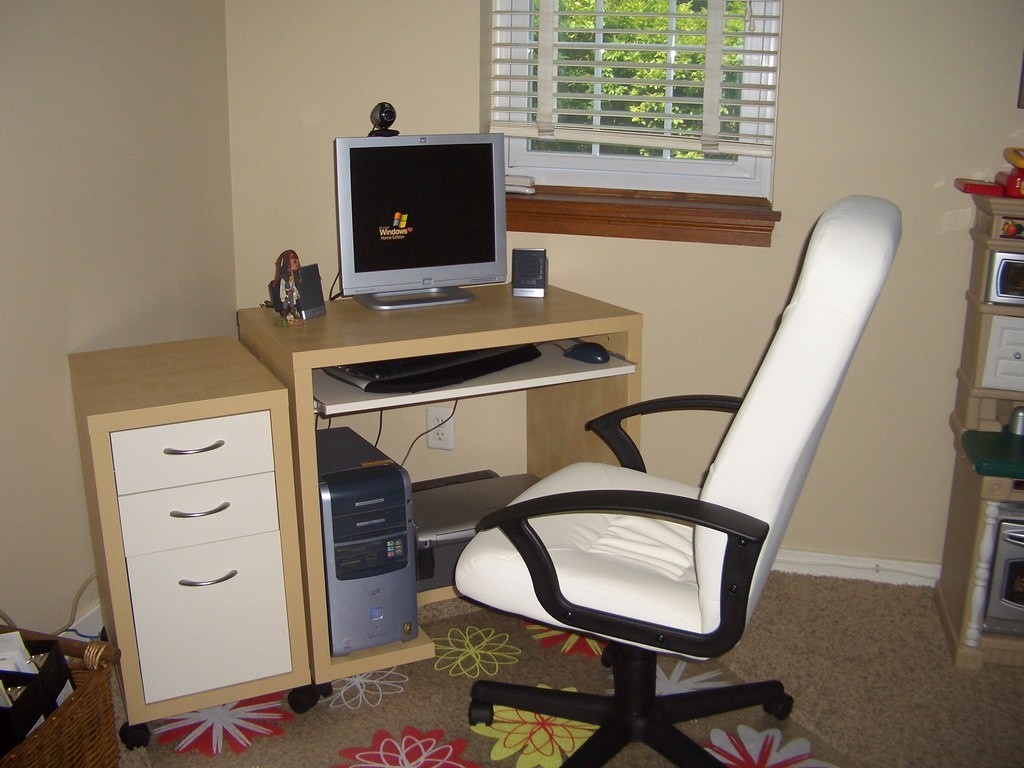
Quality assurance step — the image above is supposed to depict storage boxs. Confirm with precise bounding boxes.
[0,639,80,761]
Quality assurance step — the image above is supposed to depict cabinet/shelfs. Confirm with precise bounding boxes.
[68,334,311,750]
[933,172,1024,671]
[239,283,643,697]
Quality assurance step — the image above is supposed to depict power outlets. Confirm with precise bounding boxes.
[426,407,453,450]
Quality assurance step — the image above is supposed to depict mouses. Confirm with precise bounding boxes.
[563,342,611,364]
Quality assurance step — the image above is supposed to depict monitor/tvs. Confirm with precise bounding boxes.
[336,133,507,310]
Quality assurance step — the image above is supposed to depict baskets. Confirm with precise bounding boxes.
[0,662,120,768]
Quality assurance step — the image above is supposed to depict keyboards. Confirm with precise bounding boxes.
[323,343,542,394]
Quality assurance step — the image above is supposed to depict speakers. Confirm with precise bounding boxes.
[512,248,548,297]
[295,263,326,320]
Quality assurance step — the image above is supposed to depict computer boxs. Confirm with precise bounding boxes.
[316,426,418,657]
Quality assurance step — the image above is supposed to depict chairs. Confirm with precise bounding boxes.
[456,195,903,768]
